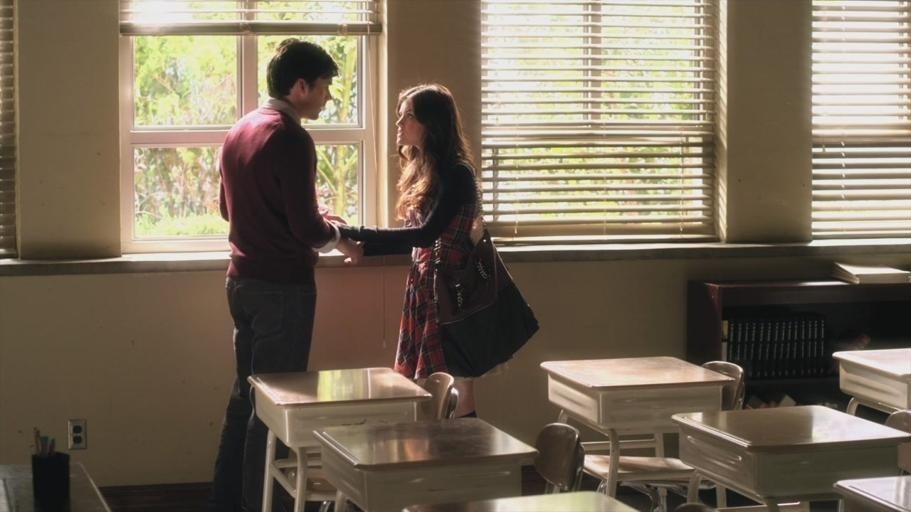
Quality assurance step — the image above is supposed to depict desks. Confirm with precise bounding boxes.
[670,405,911,511]
[832,348,910,416]
[0,460,110,511]
[832,475,911,511]
[248,367,433,512]
[400,490,641,512]
[314,418,539,512]
[539,356,736,508]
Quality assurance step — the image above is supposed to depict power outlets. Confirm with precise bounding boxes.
[68,418,87,450]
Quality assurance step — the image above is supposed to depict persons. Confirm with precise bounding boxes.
[210,38,361,509]
[332,85,539,423]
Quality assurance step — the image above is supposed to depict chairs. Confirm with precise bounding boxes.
[535,423,584,494]
[584,360,746,512]
[291,372,461,511]
[883,410,911,475]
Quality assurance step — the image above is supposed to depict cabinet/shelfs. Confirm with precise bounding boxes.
[686,277,911,431]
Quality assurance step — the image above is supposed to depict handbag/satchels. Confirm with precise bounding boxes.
[432,158,499,326]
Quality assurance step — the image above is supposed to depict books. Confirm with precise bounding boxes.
[825,262,909,283]
